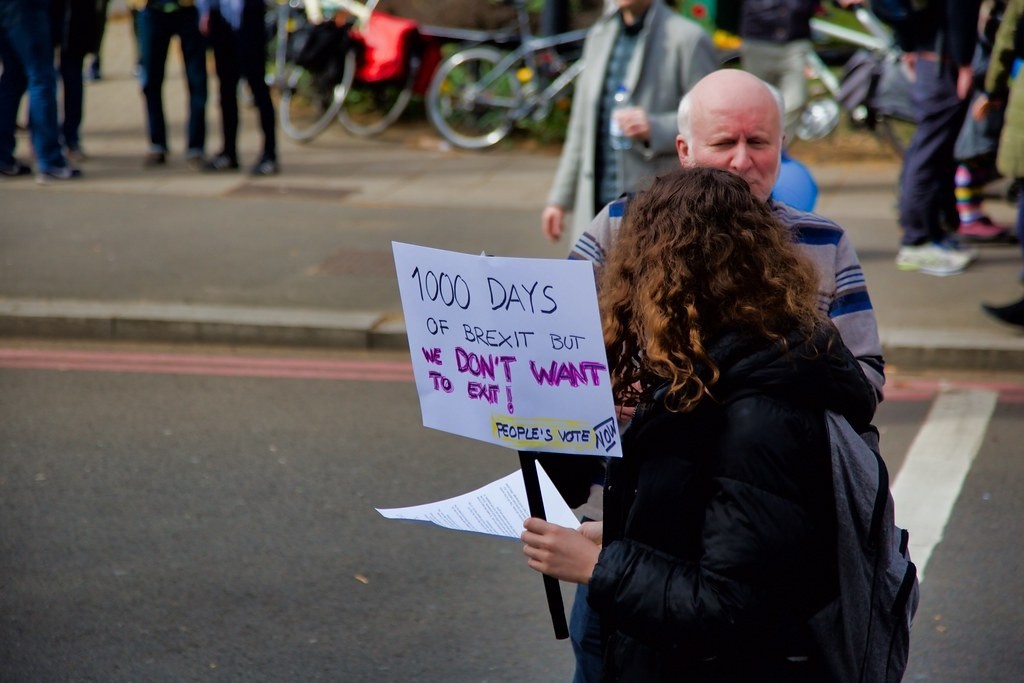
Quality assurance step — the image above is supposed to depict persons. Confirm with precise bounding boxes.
[0,0,281,184]
[490,0,1024,683]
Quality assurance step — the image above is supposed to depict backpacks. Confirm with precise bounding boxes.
[814,410,919,683]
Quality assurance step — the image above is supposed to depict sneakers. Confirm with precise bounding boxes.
[895,241,970,273]
[0,160,32,178]
[37,166,80,183]
[941,236,978,259]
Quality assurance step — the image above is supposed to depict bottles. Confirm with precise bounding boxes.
[609,86,634,152]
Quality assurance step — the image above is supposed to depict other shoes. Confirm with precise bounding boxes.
[186,153,206,170]
[87,63,98,80]
[65,147,91,164]
[981,298,1024,329]
[145,152,164,166]
[252,159,277,175]
[207,153,238,170]
[957,217,1008,238]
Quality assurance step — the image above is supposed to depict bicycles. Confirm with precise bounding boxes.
[266,1,594,151]
[800,7,924,160]
